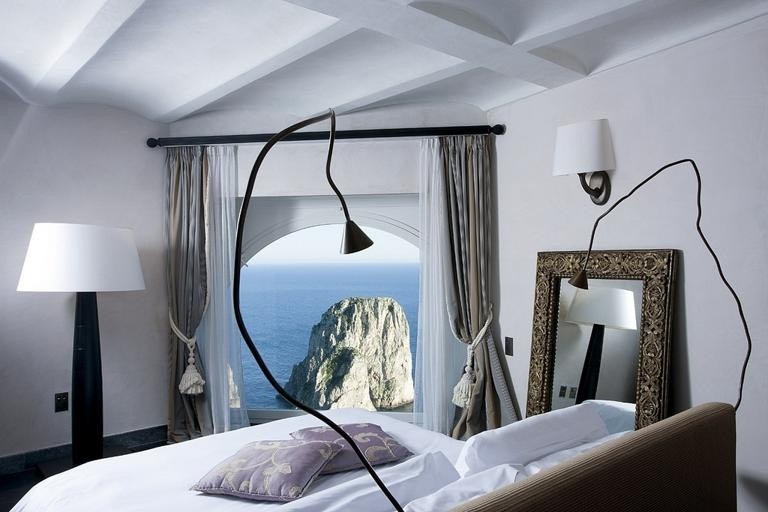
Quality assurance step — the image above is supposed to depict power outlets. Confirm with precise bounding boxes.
[54,392,69,413]
[559,385,567,397]
[569,387,577,398]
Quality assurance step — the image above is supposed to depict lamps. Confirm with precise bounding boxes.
[233,107,403,512]
[565,286,638,405]
[16,223,146,466]
[570,157,751,411]
[550,117,616,206]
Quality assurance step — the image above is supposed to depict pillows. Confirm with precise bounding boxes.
[272,451,460,512]
[291,423,413,476]
[454,401,608,477]
[522,429,634,476]
[404,463,532,512]
[187,441,343,503]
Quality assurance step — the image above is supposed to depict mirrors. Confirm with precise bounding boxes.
[526,249,679,431]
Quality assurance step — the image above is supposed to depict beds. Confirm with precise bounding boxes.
[12,401,738,511]
[583,398,636,432]
